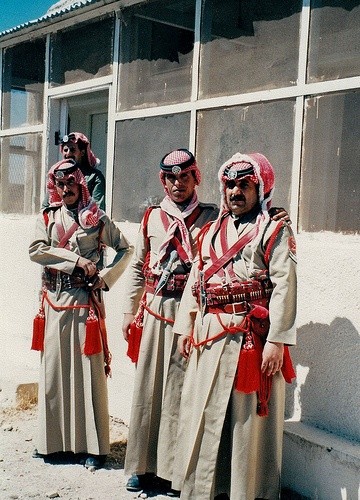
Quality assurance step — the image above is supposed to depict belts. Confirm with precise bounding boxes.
[205,298,269,315]
[146,284,183,298]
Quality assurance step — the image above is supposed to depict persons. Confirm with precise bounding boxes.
[121,147,222,492]
[41,133,105,214]
[172,152,297,500]
[28,160,134,467]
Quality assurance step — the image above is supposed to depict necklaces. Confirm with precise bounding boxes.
[69,208,78,211]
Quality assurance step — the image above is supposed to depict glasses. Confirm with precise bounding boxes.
[56,178,75,187]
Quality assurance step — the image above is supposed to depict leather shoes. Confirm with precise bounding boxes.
[31,452,41,458]
[85,456,99,468]
[125,474,141,490]
